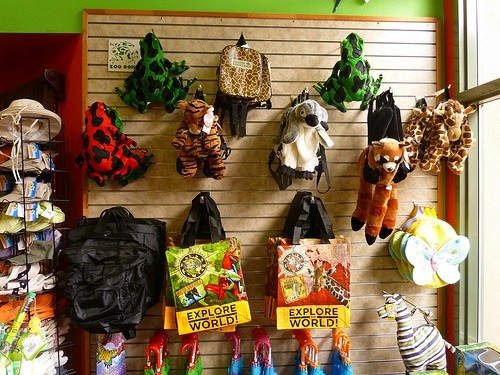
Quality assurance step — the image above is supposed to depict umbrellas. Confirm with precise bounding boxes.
[143,328,353,375]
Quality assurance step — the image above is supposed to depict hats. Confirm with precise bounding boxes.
[0,100,64,264]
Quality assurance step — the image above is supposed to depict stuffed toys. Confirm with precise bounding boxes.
[171,100,226,182]
[116,33,191,113]
[278,100,328,180]
[352,138,406,247]
[313,32,383,112]
[378,293,446,375]
[405,100,476,176]
[77,102,139,188]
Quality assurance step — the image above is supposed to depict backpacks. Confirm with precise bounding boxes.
[215,36,272,136]
[267,92,334,193]
[171,88,231,180]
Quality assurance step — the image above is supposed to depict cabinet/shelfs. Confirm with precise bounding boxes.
[0,68,76,375]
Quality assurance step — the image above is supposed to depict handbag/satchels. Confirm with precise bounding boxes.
[165,192,251,336]
[454,341,500,375]
[60,207,166,334]
[264,190,350,331]
